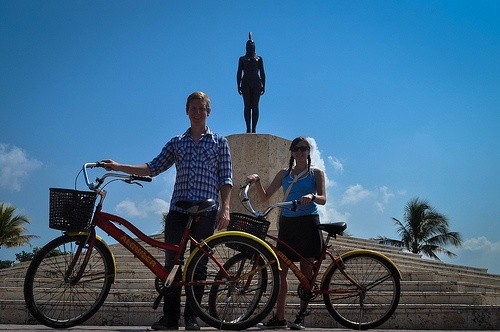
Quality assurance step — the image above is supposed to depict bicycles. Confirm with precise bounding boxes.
[23,161,283,330]
[207,177,403,330]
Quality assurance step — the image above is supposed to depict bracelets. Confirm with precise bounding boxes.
[311,193,316,201]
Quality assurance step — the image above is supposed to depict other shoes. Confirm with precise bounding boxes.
[263,317,287,328]
[291,312,305,329]
[151,315,179,329]
[185,317,200,330]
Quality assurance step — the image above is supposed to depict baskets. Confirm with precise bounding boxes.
[224,212,271,257]
[49,187,97,232]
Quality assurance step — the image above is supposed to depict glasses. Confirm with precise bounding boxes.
[289,146,308,151]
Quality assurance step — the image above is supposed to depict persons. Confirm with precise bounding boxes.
[101,92,233,330]
[247,136,326,330]
[236,32,265,133]
